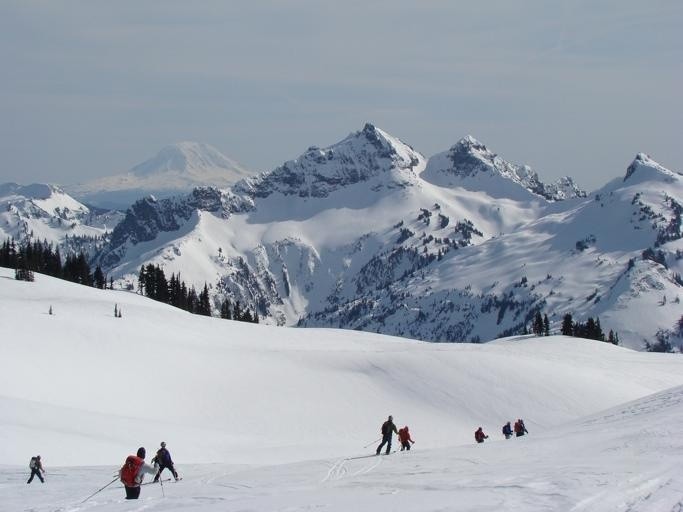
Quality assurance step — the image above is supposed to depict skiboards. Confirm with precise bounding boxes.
[145,478,187,485]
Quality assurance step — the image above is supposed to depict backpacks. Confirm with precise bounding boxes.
[120,455,144,488]
[29,457,38,469]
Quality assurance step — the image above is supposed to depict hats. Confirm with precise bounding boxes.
[137,447,145,458]
[387,415,526,442]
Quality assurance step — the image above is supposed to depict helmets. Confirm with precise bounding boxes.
[161,442,166,447]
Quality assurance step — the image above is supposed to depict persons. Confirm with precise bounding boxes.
[27,455,45,486]
[503,420,513,439]
[397,426,416,452]
[152,442,181,484]
[375,416,398,455]
[118,446,161,500]
[474,425,489,445]
[514,419,529,438]
